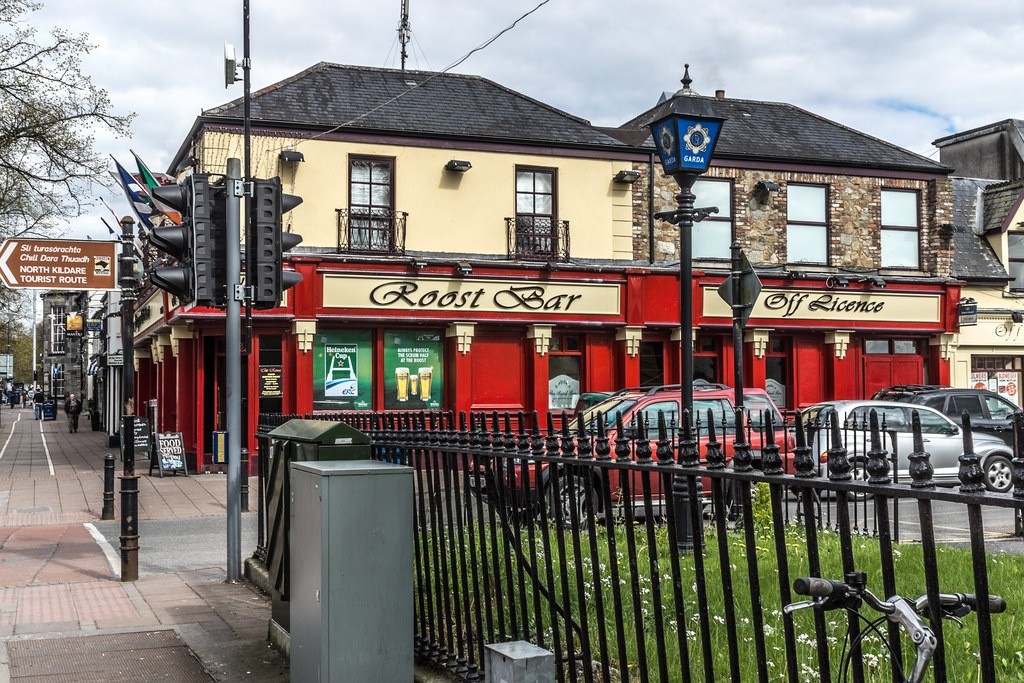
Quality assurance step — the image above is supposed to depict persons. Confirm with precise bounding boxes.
[64,394,82,434]
[4,386,44,420]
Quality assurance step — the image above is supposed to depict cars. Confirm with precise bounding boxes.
[872,384,1022,482]
[780,400,1012,503]
[467,383,799,533]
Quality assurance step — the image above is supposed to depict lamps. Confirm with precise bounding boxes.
[873,277,888,286]
[410,257,429,269]
[791,269,809,278]
[455,261,473,273]
[1011,311,1024,323]
[939,222,957,232]
[446,159,472,171]
[278,151,306,162]
[544,262,561,272]
[832,275,849,286]
[611,170,640,183]
[752,180,783,193]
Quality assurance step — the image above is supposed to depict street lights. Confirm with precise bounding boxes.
[647,59,728,555]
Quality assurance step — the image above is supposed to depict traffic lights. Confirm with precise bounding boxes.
[250,173,304,311]
[148,178,220,312]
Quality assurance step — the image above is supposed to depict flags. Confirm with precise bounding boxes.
[110,156,183,260]
